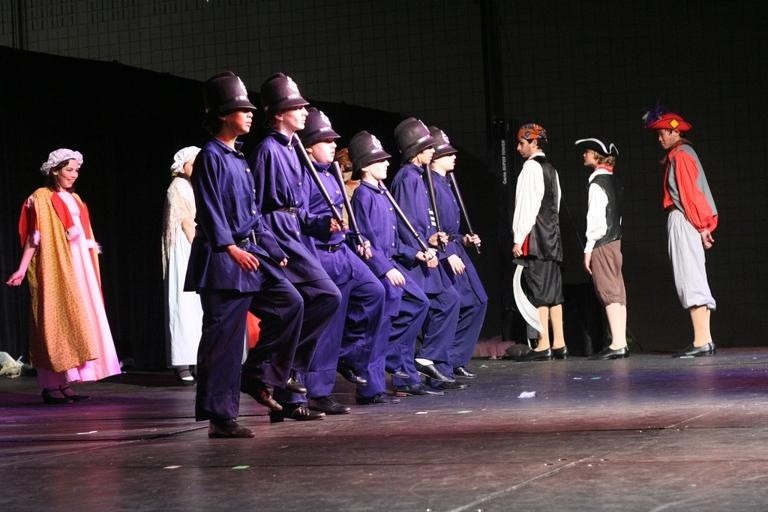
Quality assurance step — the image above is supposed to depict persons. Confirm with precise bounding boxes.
[512,123,568,362]
[5,148,122,406]
[574,131,630,360]
[642,112,720,359]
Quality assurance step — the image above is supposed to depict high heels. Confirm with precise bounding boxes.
[174,367,195,387]
[61,385,92,402]
[41,388,74,404]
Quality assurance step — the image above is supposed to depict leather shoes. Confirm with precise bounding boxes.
[239,367,285,412]
[267,404,327,420]
[286,377,307,393]
[308,394,352,414]
[334,353,478,405]
[518,346,719,360]
[208,419,255,439]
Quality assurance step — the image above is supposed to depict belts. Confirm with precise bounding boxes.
[232,239,250,248]
[447,235,456,241]
[276,206,299,216]
[316,241,343,252]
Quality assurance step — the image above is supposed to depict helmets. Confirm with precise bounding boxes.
[348,128,390,181]
[394,116,438,161]
[260,72,310,120]
[426,126,456,157]
[298,106,340,147]
[203,71,258,130]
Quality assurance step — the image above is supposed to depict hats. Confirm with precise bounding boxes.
[575,123,620,157]
[643,112,692,132]
[40,147,84,176]
[172,146,200,173]
